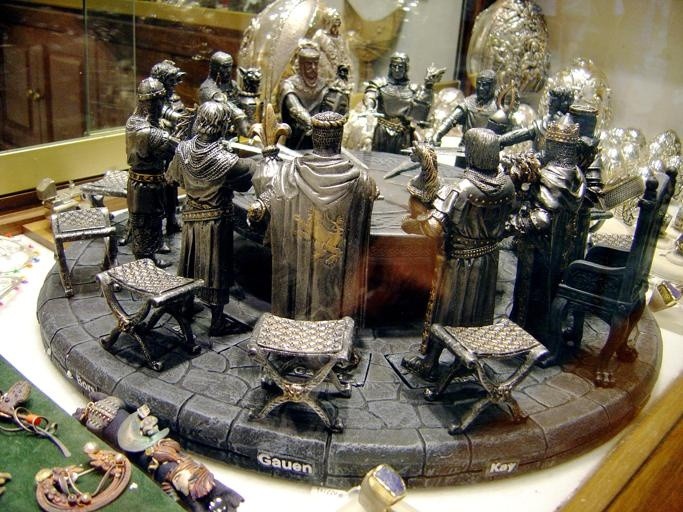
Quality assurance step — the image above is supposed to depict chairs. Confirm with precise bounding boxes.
[538,167,678,389]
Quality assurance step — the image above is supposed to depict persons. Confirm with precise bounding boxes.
[126,8,606,382]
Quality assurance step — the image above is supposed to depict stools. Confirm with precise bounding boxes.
[79,170,132,246]
[247,312,355,434]
[51,207,122,298]
[422,315,551,435]
[95,257,205,372]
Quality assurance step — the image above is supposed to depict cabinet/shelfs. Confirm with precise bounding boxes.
[0,0,259,153]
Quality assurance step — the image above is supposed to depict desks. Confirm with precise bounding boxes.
[231,149,466,325]
